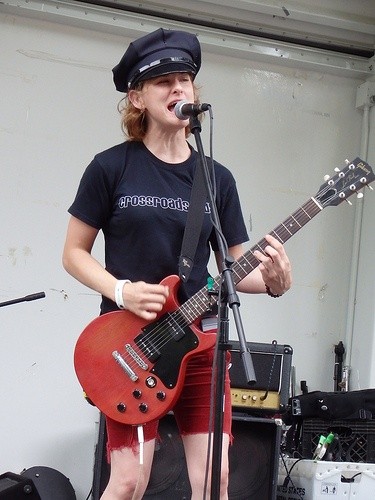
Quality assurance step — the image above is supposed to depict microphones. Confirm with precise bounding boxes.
[173,101,211,120]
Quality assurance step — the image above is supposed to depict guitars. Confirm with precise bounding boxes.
[74,156,375,428]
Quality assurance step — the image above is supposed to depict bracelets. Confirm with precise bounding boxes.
[264,284,283,297]
[115,279,130,309]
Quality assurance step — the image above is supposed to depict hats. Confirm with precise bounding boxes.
[112,27,201,93]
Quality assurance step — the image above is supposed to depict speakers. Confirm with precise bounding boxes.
[227,340,294,413]
[91,410,283,500]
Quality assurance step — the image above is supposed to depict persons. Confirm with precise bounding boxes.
[63,28,292,500]
